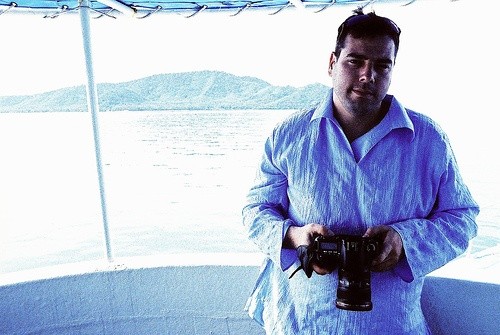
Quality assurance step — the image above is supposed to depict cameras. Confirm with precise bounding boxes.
[313,235,382,311]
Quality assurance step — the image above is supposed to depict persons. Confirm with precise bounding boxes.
[233,6,481,335]
